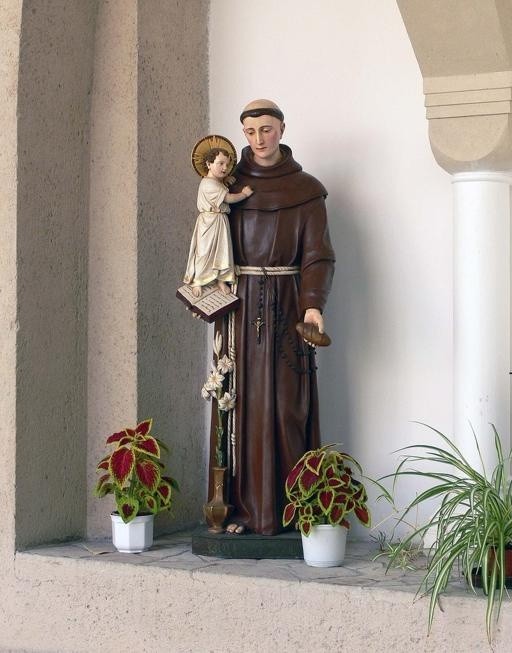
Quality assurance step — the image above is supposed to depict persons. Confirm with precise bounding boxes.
[183,148,254,300]
[185,97,337,534]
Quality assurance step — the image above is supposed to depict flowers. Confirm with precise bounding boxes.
[196,331,251,468]
[280,438,374,537]
[90,419,182,525]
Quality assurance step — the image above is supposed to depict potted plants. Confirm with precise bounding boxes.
[371,423,512,651]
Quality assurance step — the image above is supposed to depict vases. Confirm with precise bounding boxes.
[298,522,349,568]
[111,511,154,548]
[202,468,235,533]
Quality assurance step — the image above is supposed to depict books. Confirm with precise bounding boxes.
[176,280,240,322]
